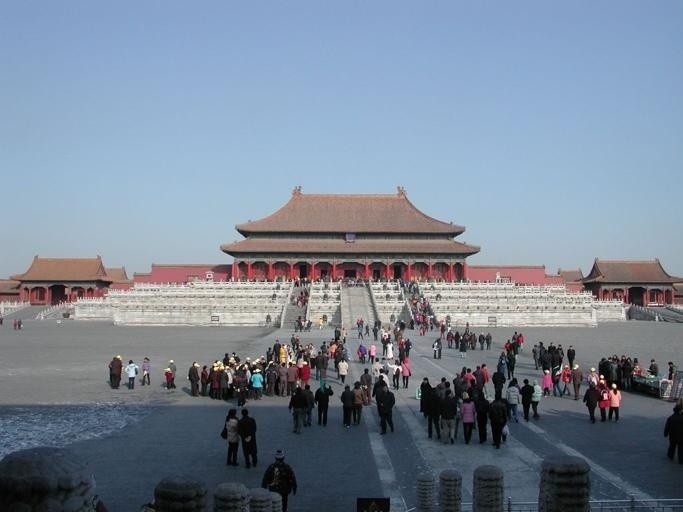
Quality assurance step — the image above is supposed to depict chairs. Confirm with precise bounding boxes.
[343,423,350,429]
[227,462,252,468]
[450,438,455,444]
[379,428,395,435]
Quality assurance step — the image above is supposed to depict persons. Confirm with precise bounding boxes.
[14,319,18,330]
[107,272,683,468]
[262,449,297,512]
[18,319,21,329]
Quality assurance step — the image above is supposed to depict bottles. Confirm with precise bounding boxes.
[163,368,170,371]
[532,379,538,384]
[611,383,617,389]
[273,449,284,460]
[192,352,310,374]
[543,369,549,373]
[590,367,596,371]
[167,359,174,363]
[599,375,605,385]
[573,364,579,369]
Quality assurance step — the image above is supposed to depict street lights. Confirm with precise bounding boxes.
[500,424,509,442]
[408,369,411,376]
[220,428,228,440]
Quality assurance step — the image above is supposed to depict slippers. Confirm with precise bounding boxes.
[599,389,608,400]
[394,367,400,377]
